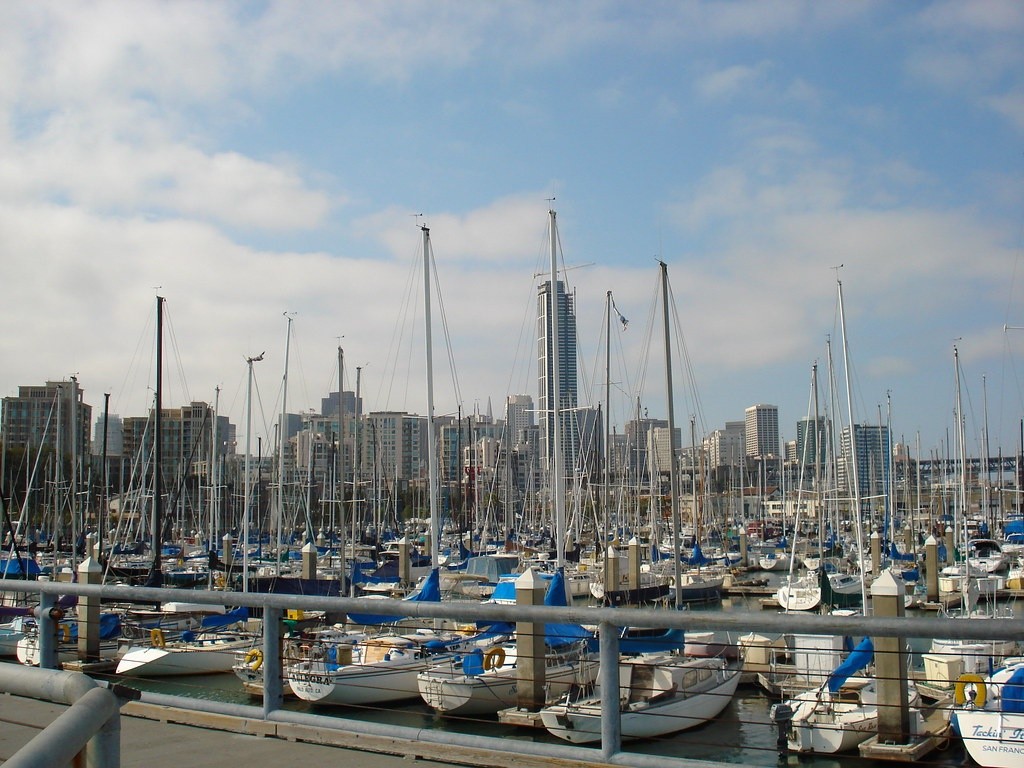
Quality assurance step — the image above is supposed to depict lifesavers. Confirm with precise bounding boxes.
[482,647,506,670]
[151,629,165,648]
[954,674,987,708]
[58,624,70,642]
[245,649,263,671]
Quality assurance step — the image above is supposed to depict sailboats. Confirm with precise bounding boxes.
[0,194,1024,767]
